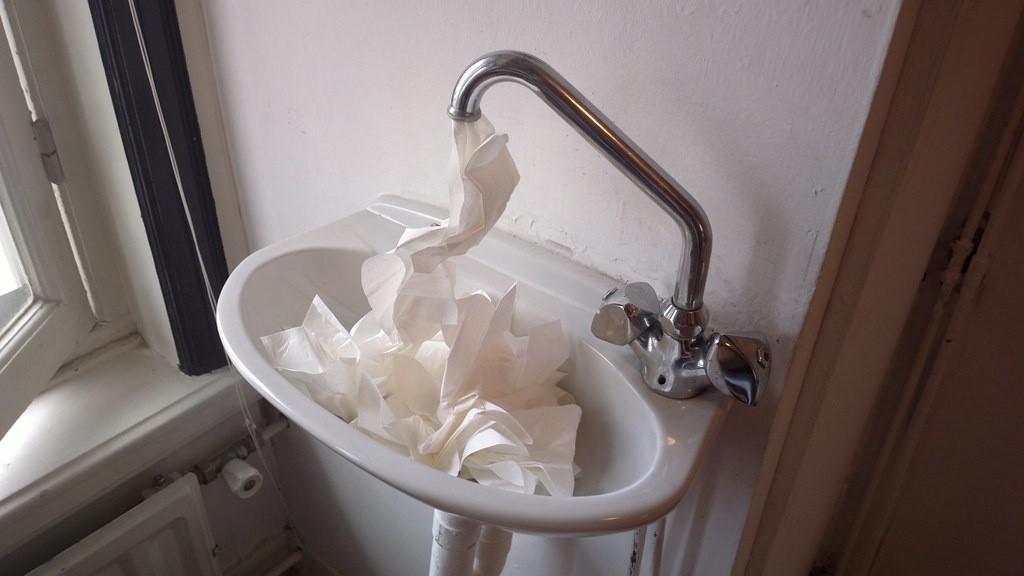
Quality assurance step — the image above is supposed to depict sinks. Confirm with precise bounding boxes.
[215,204,732,540]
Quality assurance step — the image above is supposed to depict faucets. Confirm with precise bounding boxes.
[443,50,711,329]
